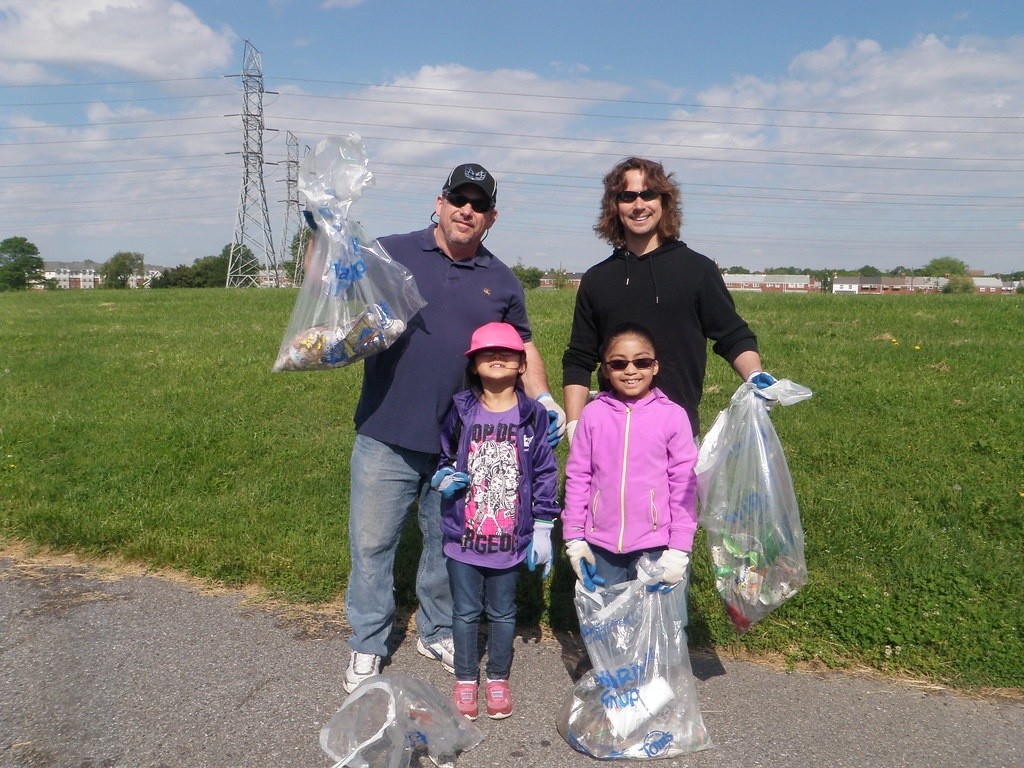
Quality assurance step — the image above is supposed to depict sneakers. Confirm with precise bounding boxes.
[417,637,456,675]
[453,679,478,721]
[342,648,381,693]
[484,677,513,719]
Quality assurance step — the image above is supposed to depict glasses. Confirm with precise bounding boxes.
[617,188,658,202]
[442,191,494,213]
[604,357,655,370]
[476,347,522,356]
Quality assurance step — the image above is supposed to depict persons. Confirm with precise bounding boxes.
[304,163,567,695]
[561,322,697,607]
[559,154,778,680]
[429,320,561,721]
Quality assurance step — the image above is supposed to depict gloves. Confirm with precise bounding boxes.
[303,188,335,231]
[536,393,566,450]
[746,371,778,414]
[646,548,689,593]
[431,467,470,500]
[523,518,554,578]
[565,539,605,592]
[567,420,578,447]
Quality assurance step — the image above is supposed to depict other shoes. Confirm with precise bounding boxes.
[573,655,593,680]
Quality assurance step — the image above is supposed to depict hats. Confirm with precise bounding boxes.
[442,163,498,208]
[465,322,526,357]
